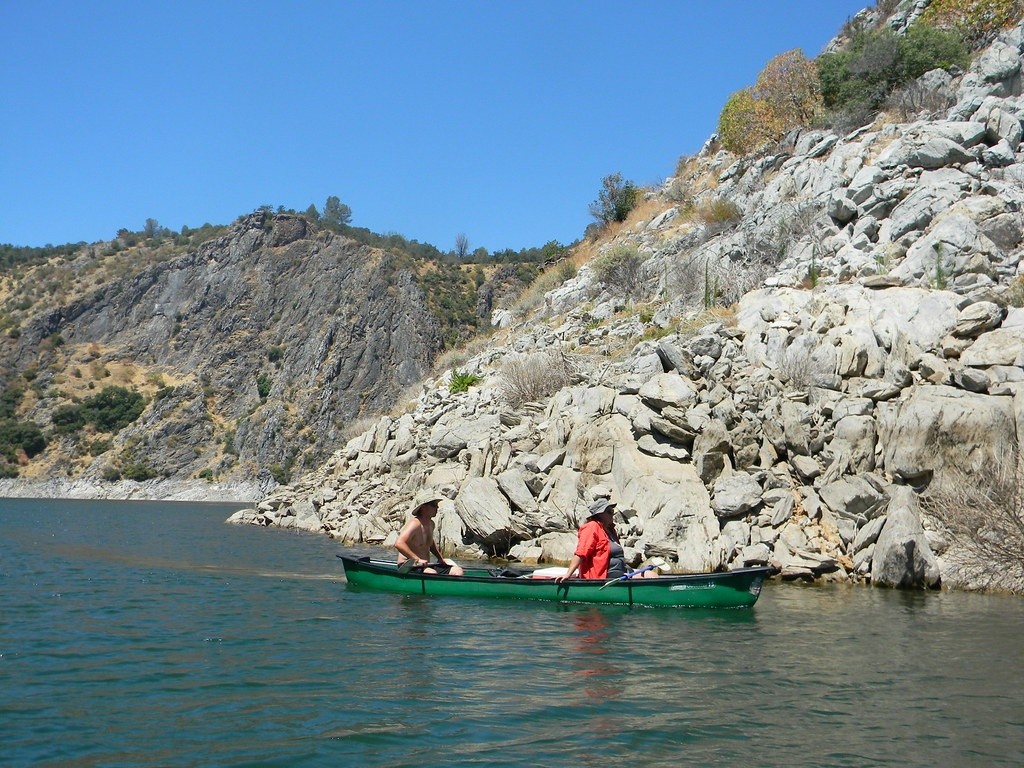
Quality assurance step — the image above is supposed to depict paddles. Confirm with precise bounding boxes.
[396,558,476,575]
[598,557,672,591]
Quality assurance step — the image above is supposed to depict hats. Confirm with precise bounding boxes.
[586,498,617,519]
[412,488,443,515]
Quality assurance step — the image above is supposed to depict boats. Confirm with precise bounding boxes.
[335,553,782,612]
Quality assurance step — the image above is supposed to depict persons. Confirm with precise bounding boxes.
[554,484,625,583]
[395,493,463,578]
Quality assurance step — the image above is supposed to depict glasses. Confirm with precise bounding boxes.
[427,502,439,508]
[605,509,614,515]
